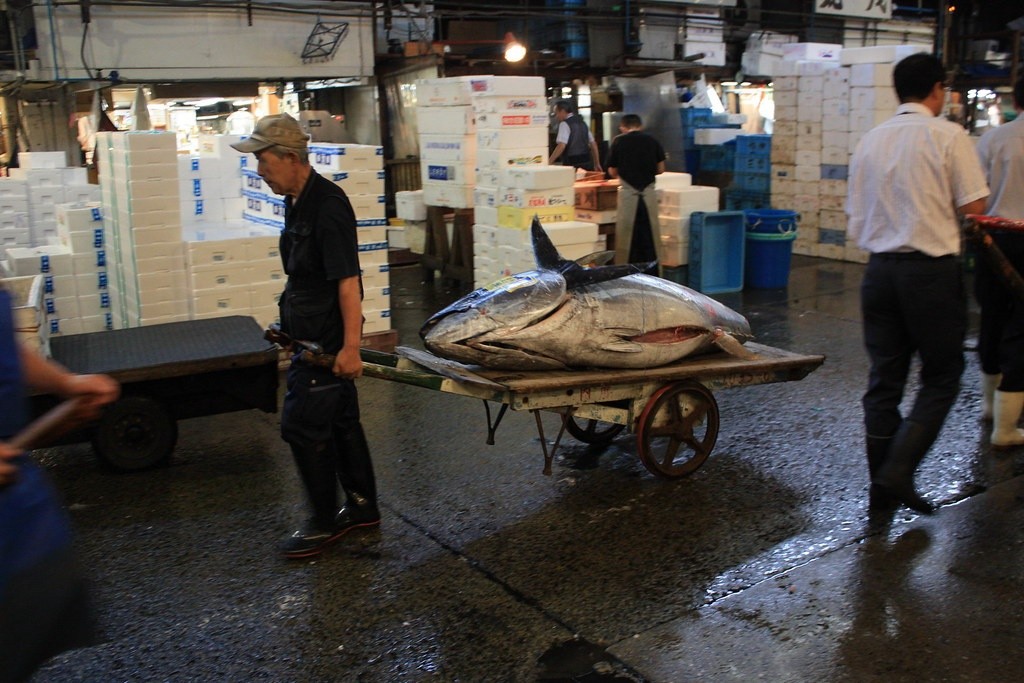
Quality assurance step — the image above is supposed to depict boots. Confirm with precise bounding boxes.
[875,420,935,516]
[990,387,1024,443]
[979,371,1002,419]
[865,432,896,525]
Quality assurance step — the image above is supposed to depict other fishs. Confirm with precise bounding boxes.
[418,212,754,371]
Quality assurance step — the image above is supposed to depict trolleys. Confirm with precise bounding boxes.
[263,320,826,487]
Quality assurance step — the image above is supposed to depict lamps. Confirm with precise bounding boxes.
[503,31,527,63]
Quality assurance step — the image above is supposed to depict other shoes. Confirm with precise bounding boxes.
[278,498,381,556]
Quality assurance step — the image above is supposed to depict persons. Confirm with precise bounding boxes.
[230,113,385,556]
[973,74,1024,446]
[604,114,666,277]
[846,52,990,530]
[548,101,603,171]
[0,288,118,683]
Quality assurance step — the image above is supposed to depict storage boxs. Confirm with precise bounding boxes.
[385,75,620,293]
[0,131,391,360]
[635,2,950,294]
[542,0,590,59]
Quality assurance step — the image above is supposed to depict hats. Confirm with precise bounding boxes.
[229,113,310,153]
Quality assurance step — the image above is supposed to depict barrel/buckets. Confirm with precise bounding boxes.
[742,206,800,289]
